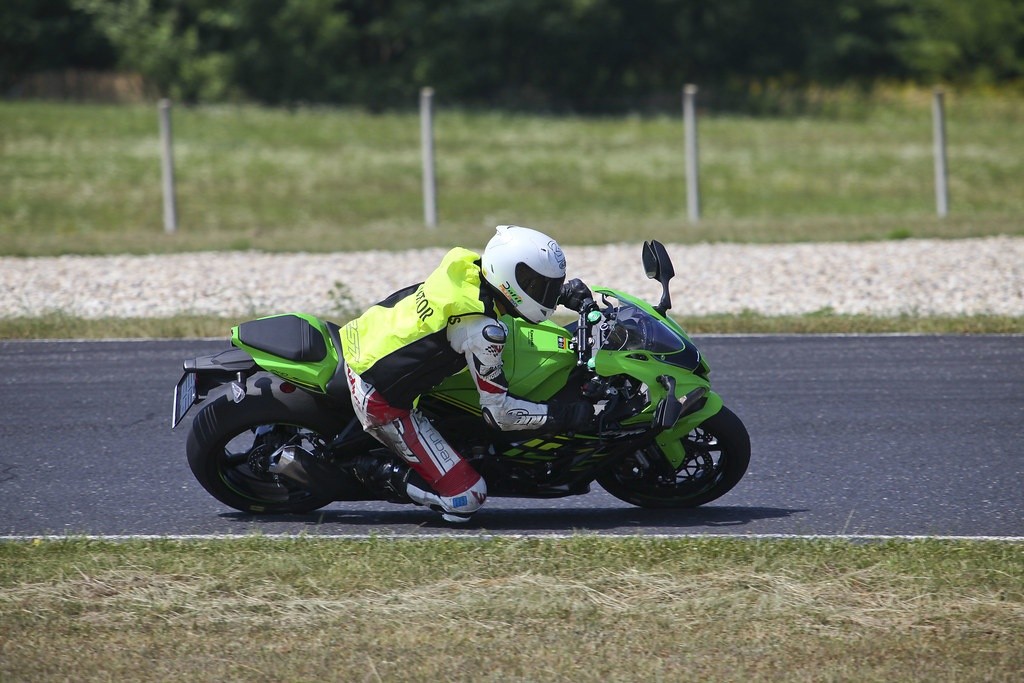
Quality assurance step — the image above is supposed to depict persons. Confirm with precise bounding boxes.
[339,225,593,523]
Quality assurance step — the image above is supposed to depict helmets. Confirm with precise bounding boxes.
[482,224,567,325]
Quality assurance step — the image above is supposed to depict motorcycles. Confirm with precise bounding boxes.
[173,239,752,518]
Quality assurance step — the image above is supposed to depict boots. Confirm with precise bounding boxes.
[347,442,424,506]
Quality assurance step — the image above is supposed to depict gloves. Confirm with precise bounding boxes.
[556,277,594,312]
[535,398,595,433]
